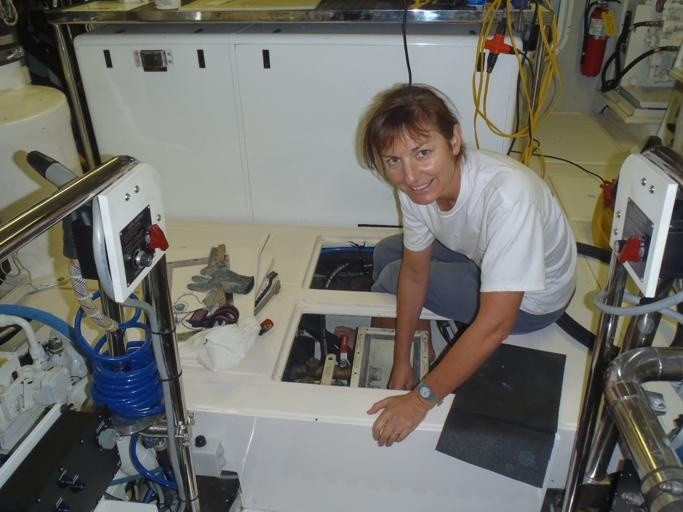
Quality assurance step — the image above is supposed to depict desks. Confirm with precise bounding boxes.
[42,1,553,227]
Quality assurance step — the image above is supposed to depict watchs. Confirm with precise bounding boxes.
[414,382,443,407]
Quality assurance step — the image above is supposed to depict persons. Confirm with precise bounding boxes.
[354,78,580,447]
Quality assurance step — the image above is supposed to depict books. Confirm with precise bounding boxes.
[599,85,680,127]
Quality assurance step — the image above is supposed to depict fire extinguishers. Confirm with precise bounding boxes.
[581,0,621,76]
